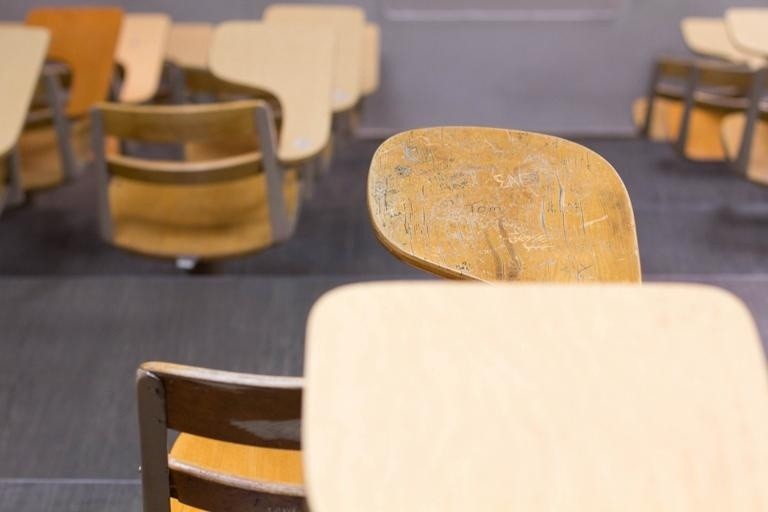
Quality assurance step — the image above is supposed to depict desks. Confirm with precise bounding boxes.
[303,277,768,510]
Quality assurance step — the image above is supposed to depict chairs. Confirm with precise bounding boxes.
[632,0,768,195]
[0,3,385,274]
[132,354,313,511]
